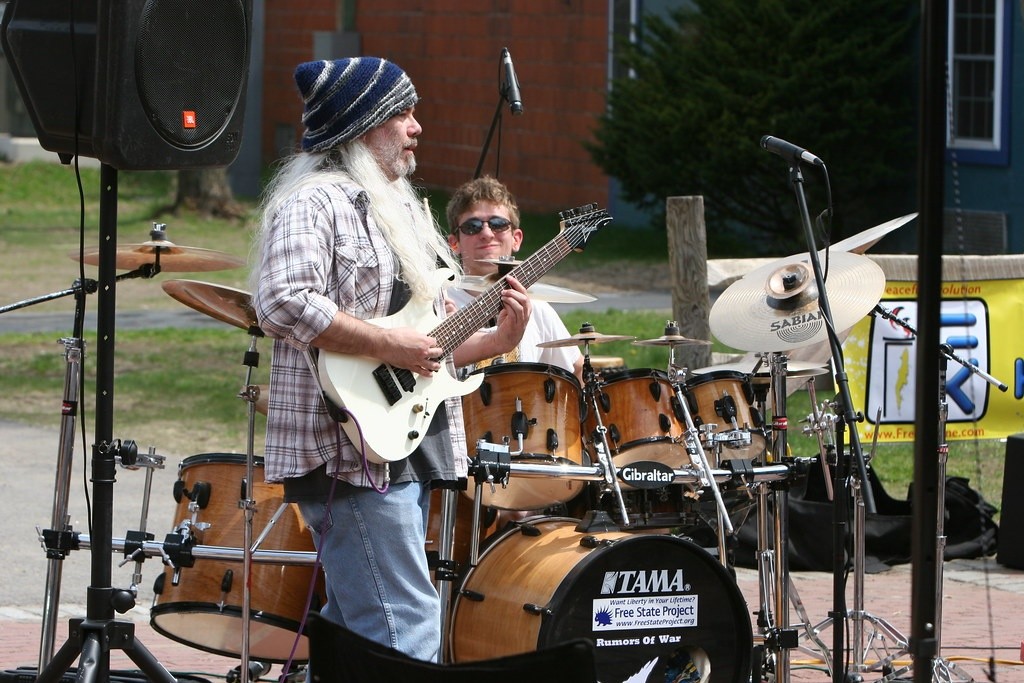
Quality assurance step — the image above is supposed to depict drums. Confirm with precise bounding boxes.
[425,478,500,595]
[149,452,326,662]
[580,369,698,491]
[675,370,767,468]
[459,363,589,510]
[447,513,753,683]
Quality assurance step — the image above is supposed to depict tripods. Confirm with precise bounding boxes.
[33,162,182,683]
[751,273,973,683]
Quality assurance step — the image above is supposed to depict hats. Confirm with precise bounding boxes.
[294,57,419,154]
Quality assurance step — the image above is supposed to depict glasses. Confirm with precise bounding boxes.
[453,217,517,237]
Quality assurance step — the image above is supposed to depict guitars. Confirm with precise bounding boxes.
[319,202,615,466]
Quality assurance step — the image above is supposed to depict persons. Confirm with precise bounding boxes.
[253,56,533,683]
[447,175,585,389]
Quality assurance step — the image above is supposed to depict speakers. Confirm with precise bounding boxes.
[0,0,254,170]
[996,433,1023,571]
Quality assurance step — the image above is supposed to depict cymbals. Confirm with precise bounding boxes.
[450,275,598,304]
[535,333,636,348]
[819,210,920,253]
[691,359,830,383]
[66,239,248,274]
[739,326,853,413]
[708,250,886,353]
[629,335,715,348]
[161,278,263,336]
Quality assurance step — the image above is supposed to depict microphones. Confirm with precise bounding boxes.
[760,134,824,166]
[502,47,523,117]
